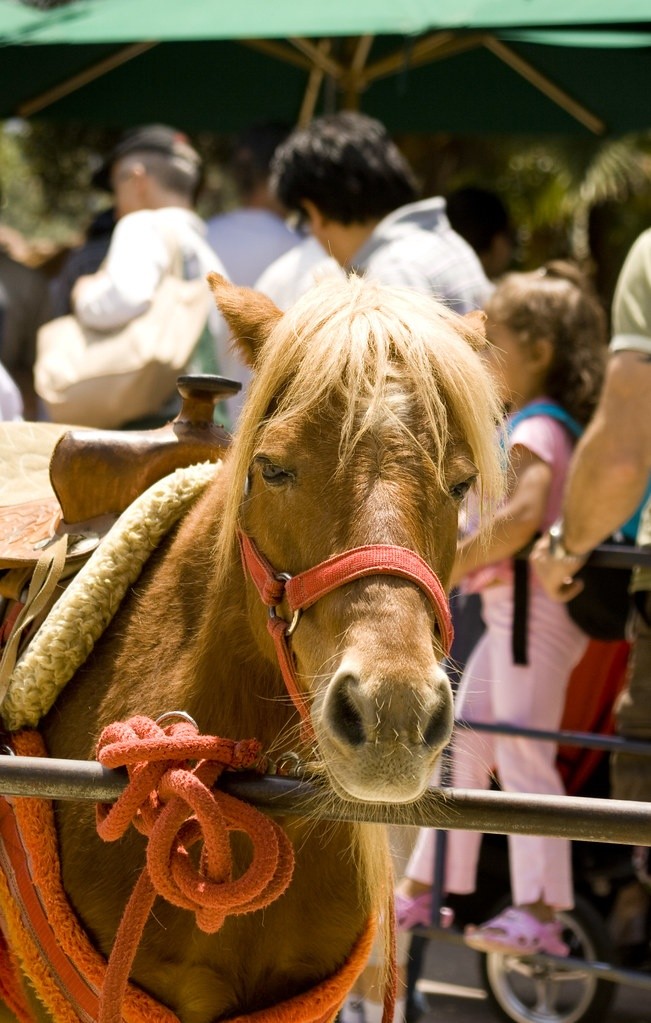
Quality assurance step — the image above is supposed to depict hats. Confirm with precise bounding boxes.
[89,123,208,192]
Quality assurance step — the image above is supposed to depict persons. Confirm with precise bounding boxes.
[0,110,651,839]
[386,254,609,958]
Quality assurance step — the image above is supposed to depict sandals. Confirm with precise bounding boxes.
[395,891,453,933]
[463,906,569,958]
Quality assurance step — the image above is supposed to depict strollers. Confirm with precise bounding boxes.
[390,527,651,1023]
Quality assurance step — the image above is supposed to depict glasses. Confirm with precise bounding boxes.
[287,210,313,237]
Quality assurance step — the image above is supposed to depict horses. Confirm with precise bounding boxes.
[0,270,514,1021]
